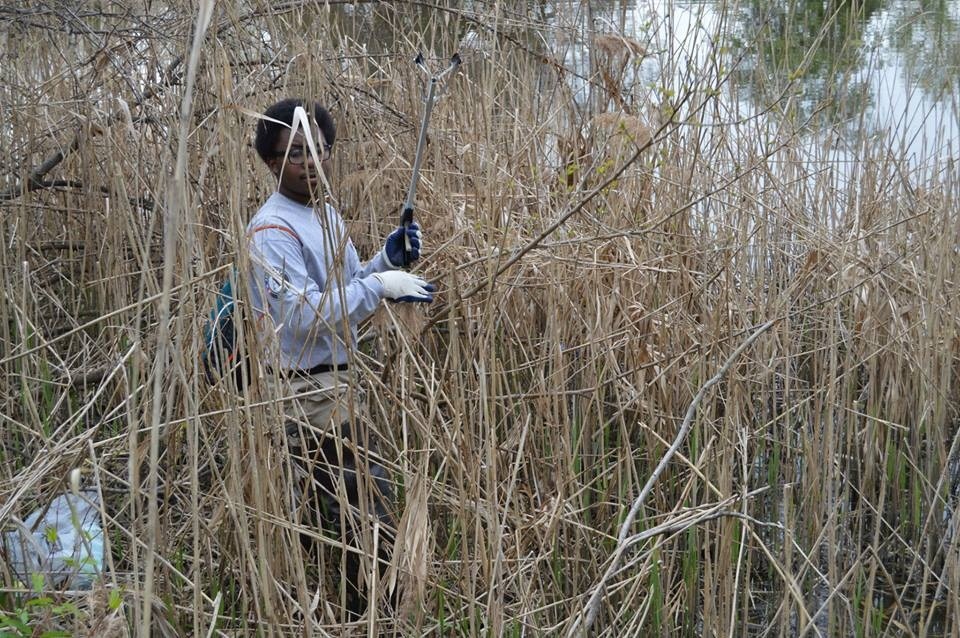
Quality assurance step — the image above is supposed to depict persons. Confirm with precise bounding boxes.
[235,97,435,638]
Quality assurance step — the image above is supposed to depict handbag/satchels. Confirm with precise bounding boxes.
[5,468,104,596]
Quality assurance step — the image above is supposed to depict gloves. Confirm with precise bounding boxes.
[376,270,436,303]
[382,222,422,267]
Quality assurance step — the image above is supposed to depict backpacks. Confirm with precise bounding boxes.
[205,226,304,393]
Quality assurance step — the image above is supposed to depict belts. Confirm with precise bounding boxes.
[266,364,348,379]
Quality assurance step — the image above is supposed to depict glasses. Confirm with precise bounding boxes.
[270,145,332,165]
